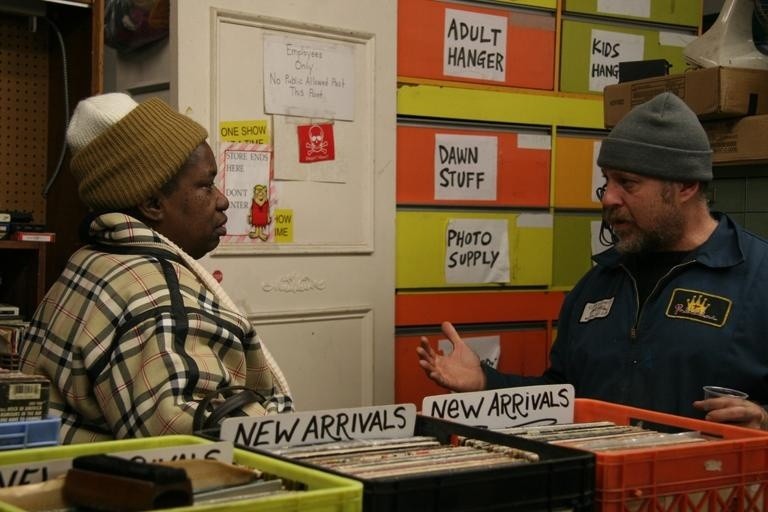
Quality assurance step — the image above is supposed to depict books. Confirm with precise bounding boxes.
[0,222,48,233]
[0,302,53,422]
[0,210,33,223]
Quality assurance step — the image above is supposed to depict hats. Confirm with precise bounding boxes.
[597,92,714,181]
[64,91,208,214]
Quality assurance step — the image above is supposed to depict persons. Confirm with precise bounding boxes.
[415,91,767,438]
[15,90,297,445]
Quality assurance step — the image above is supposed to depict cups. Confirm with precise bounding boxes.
[700,384,749,401]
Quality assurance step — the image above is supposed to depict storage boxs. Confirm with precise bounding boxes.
[394,0,618,412]
[618,0,703,76]
[707,166,768,240]
